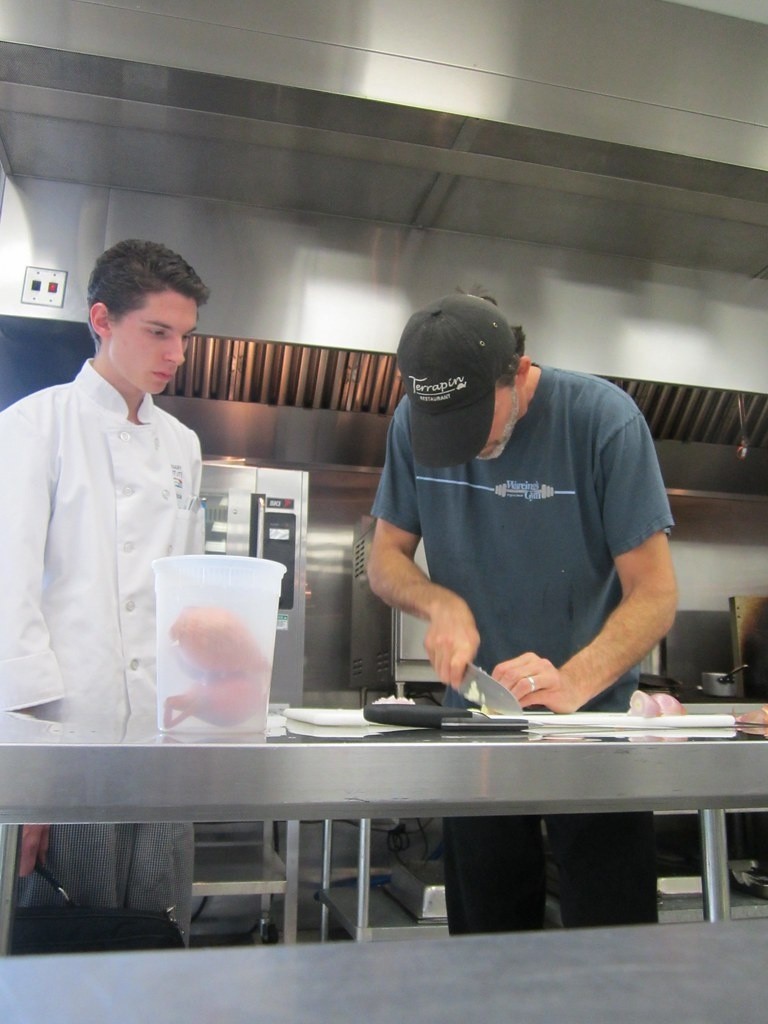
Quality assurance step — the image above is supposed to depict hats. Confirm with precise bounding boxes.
[397,293,519,469]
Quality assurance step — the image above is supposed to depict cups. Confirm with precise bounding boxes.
[153,554,287,744]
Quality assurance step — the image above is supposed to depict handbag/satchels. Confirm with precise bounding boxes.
[9,864,186,954]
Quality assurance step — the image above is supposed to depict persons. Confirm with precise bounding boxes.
[1,238,206,955]
[367,284,677,936]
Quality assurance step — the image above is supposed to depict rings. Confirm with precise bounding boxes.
[528,676,535,693]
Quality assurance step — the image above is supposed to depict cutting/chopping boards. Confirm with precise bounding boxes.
[488,715,736,727]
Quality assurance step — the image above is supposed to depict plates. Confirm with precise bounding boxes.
[283,709,385,725]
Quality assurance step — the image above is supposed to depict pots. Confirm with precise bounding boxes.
[701,665,748,696]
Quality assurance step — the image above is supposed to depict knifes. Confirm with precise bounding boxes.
[458,662,523,717]
[441,717,681,733]
